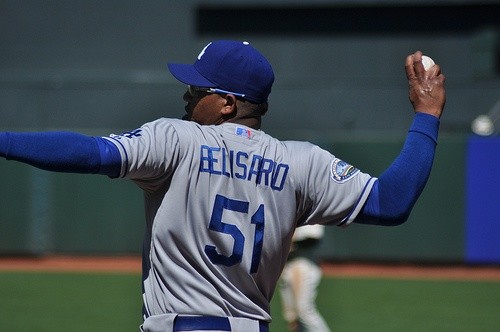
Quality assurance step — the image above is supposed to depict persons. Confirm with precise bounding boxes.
[281,224,330,332]
[1,40,446,332]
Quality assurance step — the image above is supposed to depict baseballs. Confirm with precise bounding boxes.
[421,56,435,72]
